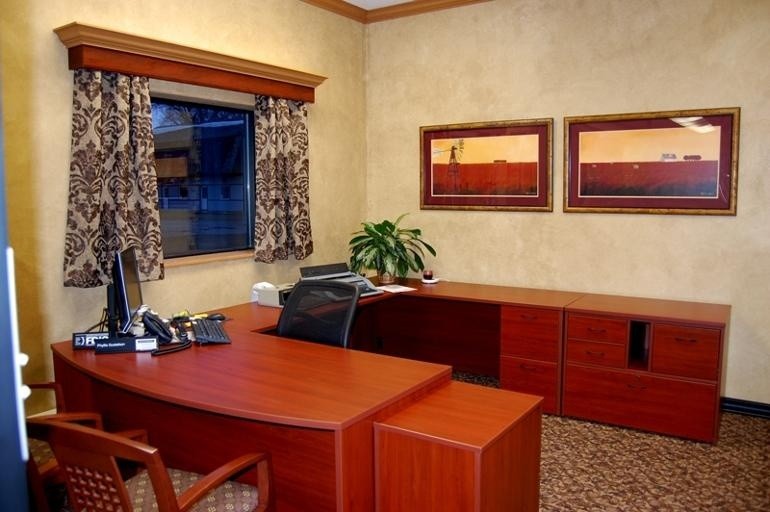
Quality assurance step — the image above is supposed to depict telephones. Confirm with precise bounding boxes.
[141,310,193,355]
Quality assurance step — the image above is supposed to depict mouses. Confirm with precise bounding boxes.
[207,312,226,320]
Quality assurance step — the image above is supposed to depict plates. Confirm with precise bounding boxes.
[421,278,441,284]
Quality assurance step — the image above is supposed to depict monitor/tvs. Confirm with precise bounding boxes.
[106,245,143,339]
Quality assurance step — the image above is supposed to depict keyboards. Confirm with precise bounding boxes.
[190,318,232,345]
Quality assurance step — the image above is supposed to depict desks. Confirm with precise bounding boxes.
[48,274,584,512]
[564,294,733,446]
[374,378,546,512]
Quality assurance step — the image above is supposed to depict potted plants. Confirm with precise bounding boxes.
[348,212,437,284]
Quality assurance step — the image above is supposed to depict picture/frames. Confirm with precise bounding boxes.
[560,107,742,217]
[418,117,555,213]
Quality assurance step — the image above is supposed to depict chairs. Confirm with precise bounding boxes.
[18,421,273,512]
[20,376,98,425]
[274,280,361,349]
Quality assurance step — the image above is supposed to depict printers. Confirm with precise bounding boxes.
[257,262,384,310]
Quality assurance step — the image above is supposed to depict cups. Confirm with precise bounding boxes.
[423,271,433,280]
